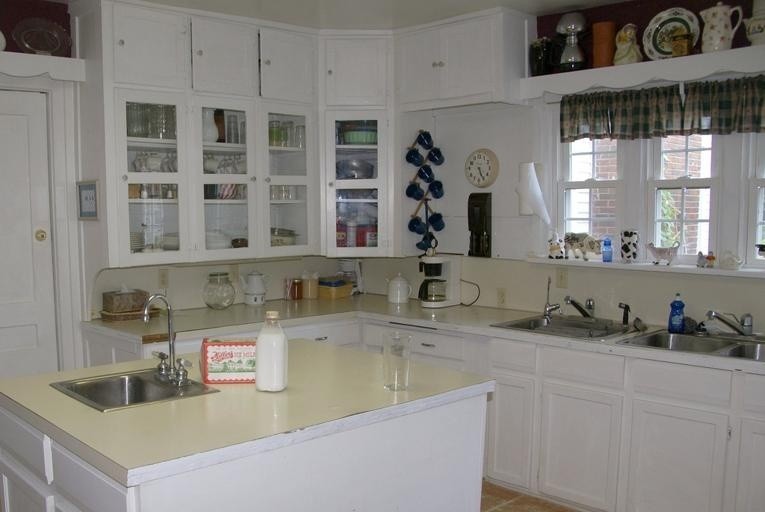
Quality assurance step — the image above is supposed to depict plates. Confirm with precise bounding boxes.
[642,6,700,60]
[130,231,145,253]
[160,233,180,250]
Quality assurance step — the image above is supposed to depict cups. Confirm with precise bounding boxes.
[296,125,305,148]
[240,121,246,144]
[282,121,294,147]
[269,120,281,147]
[227,115,239,143]
[380,331,412,392]
[620,230,639,262]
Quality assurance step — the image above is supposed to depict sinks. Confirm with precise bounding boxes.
[489,313,637,340]
[616,328,735,363]
[48,367,221,413]
[727,340,765,363]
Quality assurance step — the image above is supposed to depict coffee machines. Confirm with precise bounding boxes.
[557,12,586,71]
[419,255,463,308]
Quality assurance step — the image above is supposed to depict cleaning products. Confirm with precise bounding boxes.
[668,292,685,333]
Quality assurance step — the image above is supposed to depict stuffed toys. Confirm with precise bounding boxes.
[643,240,680,265]
[549,237,564,260]
[695,252,707,267]
[563,233,602,260]
[704,252,716,268]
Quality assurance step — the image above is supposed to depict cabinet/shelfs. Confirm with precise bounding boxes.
[321,29,392,107]
[259,20,319,106]
[102,0,192,93]
[284,311,360,344]
[361,319,464,366]
[319,110,393,260]
[616,357,731,511]
[190,95,317,261]
[143,330,271,363]
[722,368,765,512]
[458,334,537,496]
[109,91,191,261]
[191,9,260,101]
[392,6,529,113]
[0,339,495,512]
[534,344,625,512]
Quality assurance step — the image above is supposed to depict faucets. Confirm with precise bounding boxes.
[563,295,596,318]
[143,293,193,387]
[705,309,754,336]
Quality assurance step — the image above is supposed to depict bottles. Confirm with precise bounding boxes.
[151,185,164,196]
[167,183,173,198]
[292,279,303,302]
[603,238,611,262]
[346,222,355,246]
[174,186,178,198]
[255,311,289,393]
[141,184,147,198]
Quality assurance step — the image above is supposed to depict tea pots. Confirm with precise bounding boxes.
[699,1,743,54]
[384,272,413,304]
[742,10,765,45]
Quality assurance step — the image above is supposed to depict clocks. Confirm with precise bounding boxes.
[463,148,498,188]
